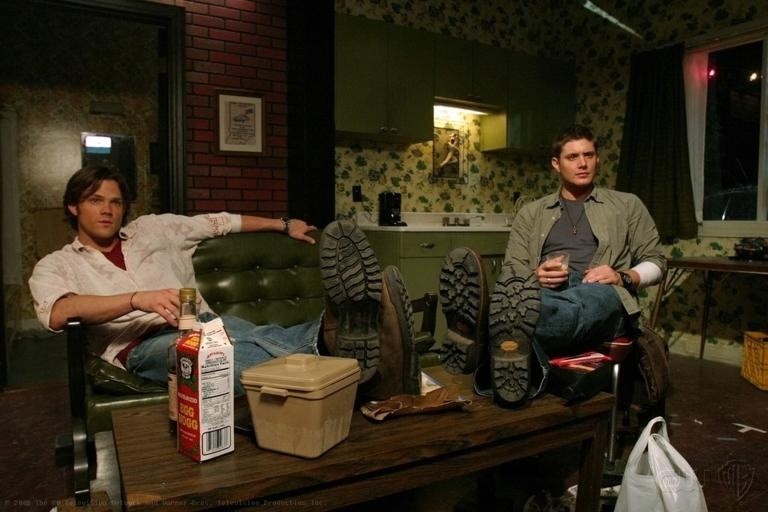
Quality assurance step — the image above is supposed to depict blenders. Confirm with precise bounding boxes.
[378,190,409,227]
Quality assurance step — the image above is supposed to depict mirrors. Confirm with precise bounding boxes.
[80,132,136,203]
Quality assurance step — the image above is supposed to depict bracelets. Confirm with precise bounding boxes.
[130,290,138,311]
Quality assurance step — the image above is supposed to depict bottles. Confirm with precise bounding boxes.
[167,287,201,438]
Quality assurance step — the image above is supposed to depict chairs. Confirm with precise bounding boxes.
[547,334,638,470]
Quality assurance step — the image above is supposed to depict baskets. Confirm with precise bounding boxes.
[740,331,768,390]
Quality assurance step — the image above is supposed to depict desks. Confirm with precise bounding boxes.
[650,256,768,360]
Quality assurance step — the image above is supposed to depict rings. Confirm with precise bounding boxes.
[547,277,551,285]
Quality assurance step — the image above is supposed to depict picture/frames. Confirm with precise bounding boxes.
[215,88,267,157]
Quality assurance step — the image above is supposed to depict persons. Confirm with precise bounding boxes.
[27,165,422,398]
[438,126,670,408]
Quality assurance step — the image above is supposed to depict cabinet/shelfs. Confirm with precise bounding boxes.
[363,232,510,351]
[334,12,576,160]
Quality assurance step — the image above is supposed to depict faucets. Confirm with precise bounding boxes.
[455,217,460,225]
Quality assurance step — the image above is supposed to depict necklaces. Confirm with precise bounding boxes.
[561,190,586,237]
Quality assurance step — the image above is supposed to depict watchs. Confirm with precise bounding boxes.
[280,216,290,234]
[618,270,633,290]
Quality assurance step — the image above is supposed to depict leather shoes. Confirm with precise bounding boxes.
[436,246,489,376]
[486,263,542,408]
[317,217,384,386]
[366,265,421,400]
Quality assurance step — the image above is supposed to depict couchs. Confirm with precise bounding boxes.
[69,228,439,512]
